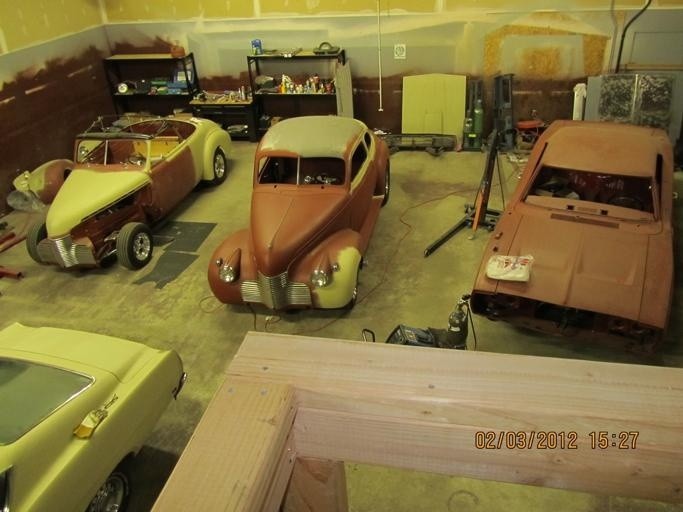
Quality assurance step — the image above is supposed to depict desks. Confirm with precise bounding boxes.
[189,93,262,142]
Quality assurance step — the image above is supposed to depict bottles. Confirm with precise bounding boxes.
[223,86,251,103]
[473,98,484,134]
[277,72,333,96]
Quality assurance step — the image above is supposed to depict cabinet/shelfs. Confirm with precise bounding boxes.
[104,53,200,120]
[246,52,346,142]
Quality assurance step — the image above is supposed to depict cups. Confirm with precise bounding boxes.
[251,39,262,55]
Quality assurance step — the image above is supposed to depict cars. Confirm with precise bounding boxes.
[205,113,392,316]
[25,109,234,268]
[1,320,186,511]
[471,117,676,364]
